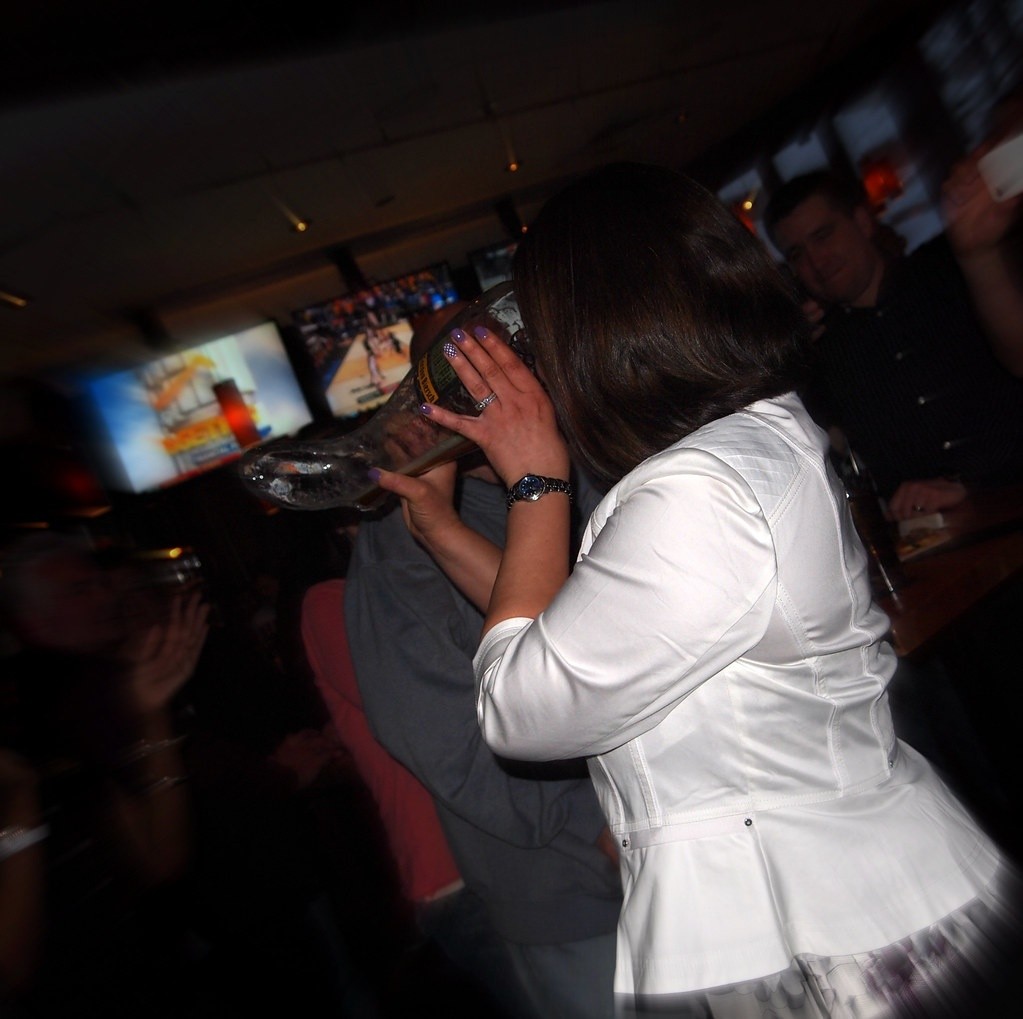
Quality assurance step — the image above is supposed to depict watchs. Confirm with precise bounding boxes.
[505,473,576,512]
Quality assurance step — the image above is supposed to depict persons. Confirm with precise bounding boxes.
[0,546,212,1019]
[365,160,1023,1019]
[167,295,609,1019]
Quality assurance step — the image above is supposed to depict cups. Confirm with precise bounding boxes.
[237,280,545,512]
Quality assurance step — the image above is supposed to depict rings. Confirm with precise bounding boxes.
[911,503,925,512]
[475,392,497,411]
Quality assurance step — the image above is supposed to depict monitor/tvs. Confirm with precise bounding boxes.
[76,235,531,503]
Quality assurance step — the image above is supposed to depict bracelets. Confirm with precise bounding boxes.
[135,729,191,760]
[138,771,193,788]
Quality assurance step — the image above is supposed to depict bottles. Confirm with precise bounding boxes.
[294,245,527,369]
[827,447,907,599]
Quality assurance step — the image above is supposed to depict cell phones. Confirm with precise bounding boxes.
[976,132,1023,202]
